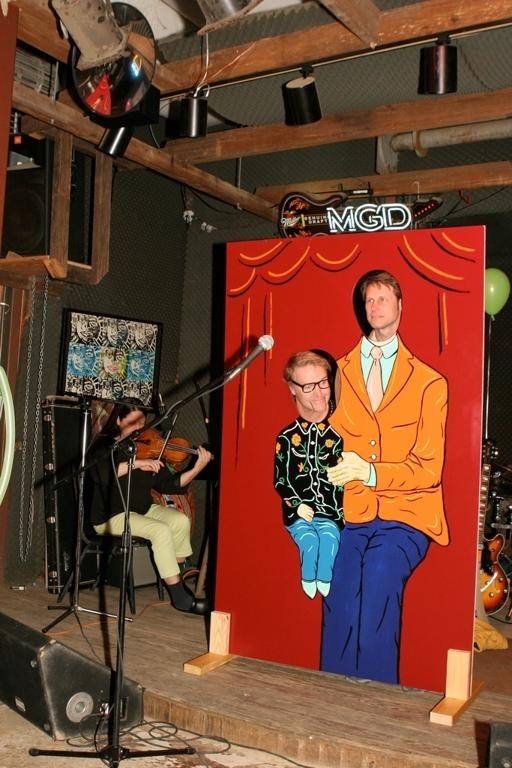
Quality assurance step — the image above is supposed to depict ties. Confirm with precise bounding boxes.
[366,347,384,410]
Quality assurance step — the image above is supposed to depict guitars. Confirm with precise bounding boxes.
[479,439,510,617]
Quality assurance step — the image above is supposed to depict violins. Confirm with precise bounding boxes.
[134,428,214,463]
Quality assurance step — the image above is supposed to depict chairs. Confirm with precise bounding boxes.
[56,460,163,618]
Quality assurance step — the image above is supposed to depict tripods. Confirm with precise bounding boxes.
[42,394,159,633]
[29,372,196,768]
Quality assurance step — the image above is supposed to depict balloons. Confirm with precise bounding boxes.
[484,267,510,322]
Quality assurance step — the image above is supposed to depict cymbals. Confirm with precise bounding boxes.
[492,460,512,479]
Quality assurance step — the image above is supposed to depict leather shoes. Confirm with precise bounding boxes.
[168,580,196,611]
[179,561,192,572]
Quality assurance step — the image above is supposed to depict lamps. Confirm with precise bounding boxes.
[281,61,322,128]
[98,117,133,157]
[178,88,210,138]
[418,31,459,96]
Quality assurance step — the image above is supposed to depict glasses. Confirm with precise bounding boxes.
[290,378,330,393]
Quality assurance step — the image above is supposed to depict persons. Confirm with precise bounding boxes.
[86,397,215,616]
[358,273,402,343]
[284,351,336,421]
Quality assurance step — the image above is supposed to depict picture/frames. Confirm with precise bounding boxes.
[60,308,163,414]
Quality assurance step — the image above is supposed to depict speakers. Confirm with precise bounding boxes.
[487,723,512,768]
[0,132,96,265]
[0,611,146,740]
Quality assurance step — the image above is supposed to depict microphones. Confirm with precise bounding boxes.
[228,334,274,381]
[157,393,170,430]
